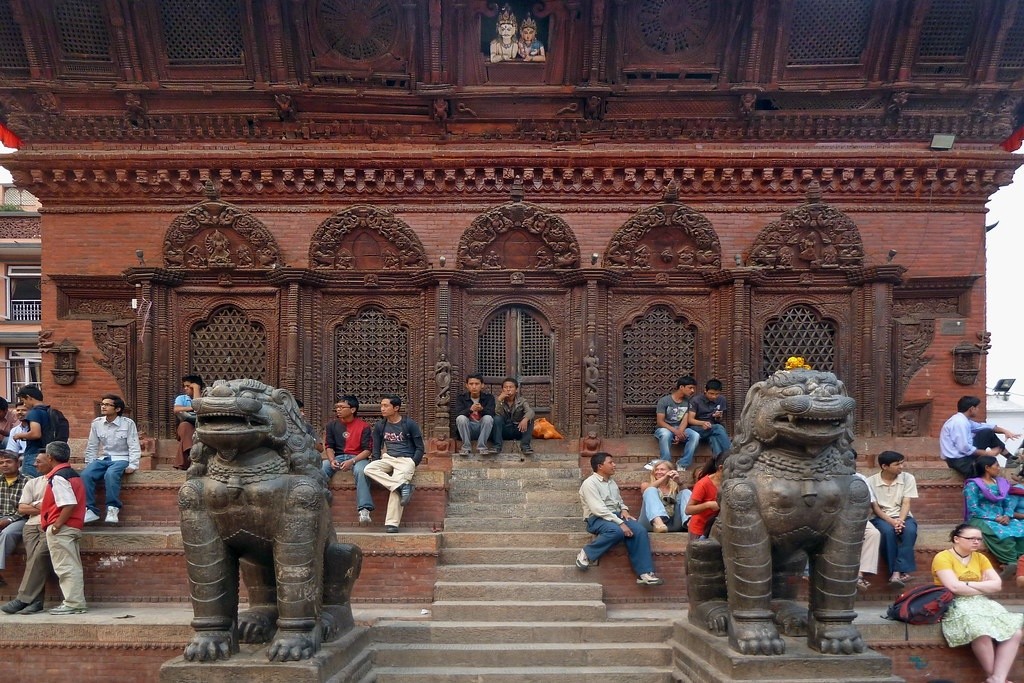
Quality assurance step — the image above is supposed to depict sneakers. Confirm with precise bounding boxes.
[15,601,44,614]
[576,548,590,570]
[83,508,100,523]
[48,603,86,615]
[103,506,120,523]
[401,485,416,505]
[2,597,24,613]
[636,572,663,587]
[358,508,371,523]
[384,526,399,533]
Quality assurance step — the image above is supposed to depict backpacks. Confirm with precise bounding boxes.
[876,586,954,625]
[45,406,69,441]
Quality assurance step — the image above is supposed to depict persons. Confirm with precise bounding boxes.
[929,522,1024,683]
[637,460,693,532]
[318,395,375,523]
[584,345,600,394]
[295,399,324,453]
[960,455,1024,571]
[0,373,205,618]
[1009,465,1024,537]
[684,451,730,596]
[688,378,731,459]
[939,395,1023,478]
[488,4,519,63]
[850,448,882,588]
[363,394,425,535]
[433,352,452,400]
[517,17,546,62]
[642,376,702,472]
[866,450,920,588]
[490,378,535,454]
[455,373,494,455]
[574,452,664,586]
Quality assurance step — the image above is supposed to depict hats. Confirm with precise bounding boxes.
[0,448,18,459]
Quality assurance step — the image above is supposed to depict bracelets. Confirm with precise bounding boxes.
[530,56,534,62]
[966,581,968,586]
[501,55,505,61]
[351,458,356,465]
[330,459,334,464]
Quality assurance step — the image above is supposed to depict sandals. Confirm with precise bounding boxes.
[887,578,905,587]
[899,574,914,582]
[862,579,871,587]
[856,577,867,591]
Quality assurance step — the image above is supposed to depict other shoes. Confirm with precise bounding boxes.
[675,461,685,471]
[522,448,534,455]
[488,447,500,453]
[999,563,1017,580]
[652,523,669,532]
[459,450,470,455]
[478,449,489,455]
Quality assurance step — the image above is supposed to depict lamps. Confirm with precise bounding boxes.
[886,249,897,263]
[993,378,1016,401]
[136,250,146,267]
[929,133,956,149]
[439,256,446,268]
[734,254,741,266]
[591,252,598,266]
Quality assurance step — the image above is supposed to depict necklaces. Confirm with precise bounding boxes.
[952,546,970,559]
[499,37,514,49]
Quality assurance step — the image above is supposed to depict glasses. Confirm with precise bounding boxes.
[956,535,984,544]
[98,401,115,407]
[334,405,351,409]
